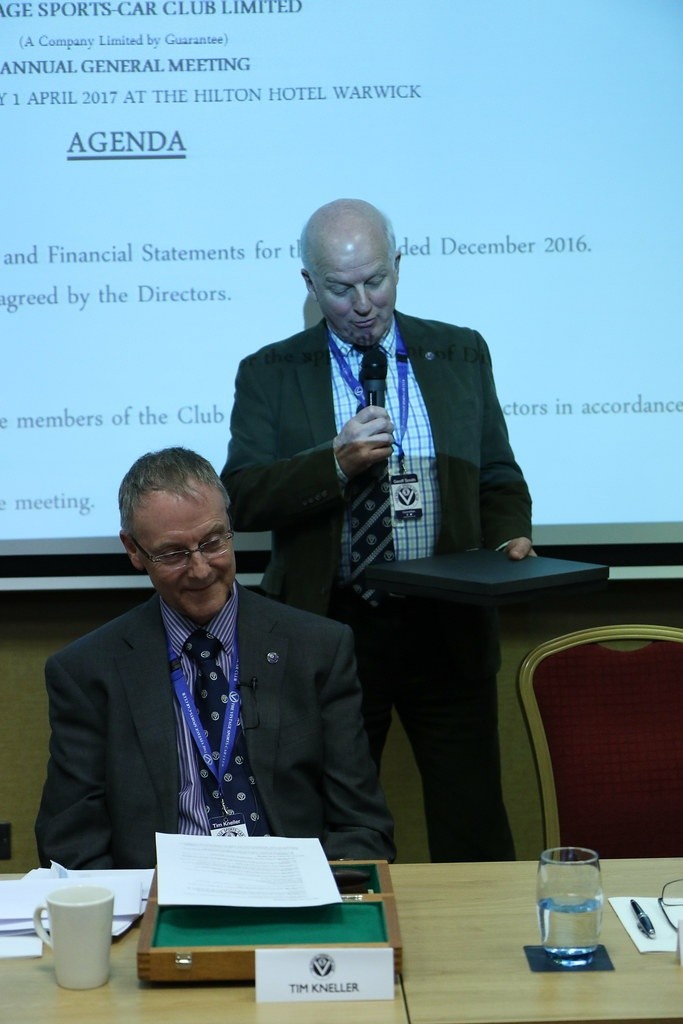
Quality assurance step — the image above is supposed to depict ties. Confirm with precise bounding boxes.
[355,345,397,609]
[181,630,273,837]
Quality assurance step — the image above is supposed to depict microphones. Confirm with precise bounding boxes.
[250,676,257,695]
[361,349,387,480]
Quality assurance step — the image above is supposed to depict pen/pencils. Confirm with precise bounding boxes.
[630,899,657,939]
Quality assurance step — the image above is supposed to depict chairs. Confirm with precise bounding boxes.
[516,625,683,860]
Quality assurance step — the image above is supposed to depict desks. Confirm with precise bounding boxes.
[0,861,410,1024]
[385,855,683,1024]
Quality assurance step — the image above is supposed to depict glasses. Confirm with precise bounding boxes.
[127,527,237,570]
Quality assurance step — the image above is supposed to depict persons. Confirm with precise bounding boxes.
[29,447,398,871]
[220,200,538,863]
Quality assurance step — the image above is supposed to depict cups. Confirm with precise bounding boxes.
[537,847,604,966]
[33,887,114,990]
